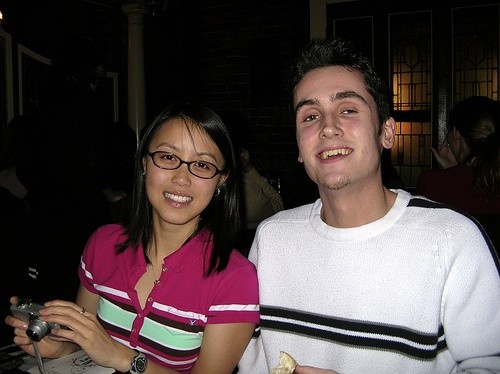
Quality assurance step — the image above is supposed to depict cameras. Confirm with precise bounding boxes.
[10,297,68,342]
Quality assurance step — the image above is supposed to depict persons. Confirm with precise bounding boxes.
[4,103,261,374]
[235,39,500,374]
[0,95,499,264]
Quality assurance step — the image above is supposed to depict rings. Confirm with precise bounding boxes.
[80,308,85,315]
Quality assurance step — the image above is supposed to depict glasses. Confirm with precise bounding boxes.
[147,150,226,179]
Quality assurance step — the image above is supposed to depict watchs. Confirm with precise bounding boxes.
[115,348,147,374]
